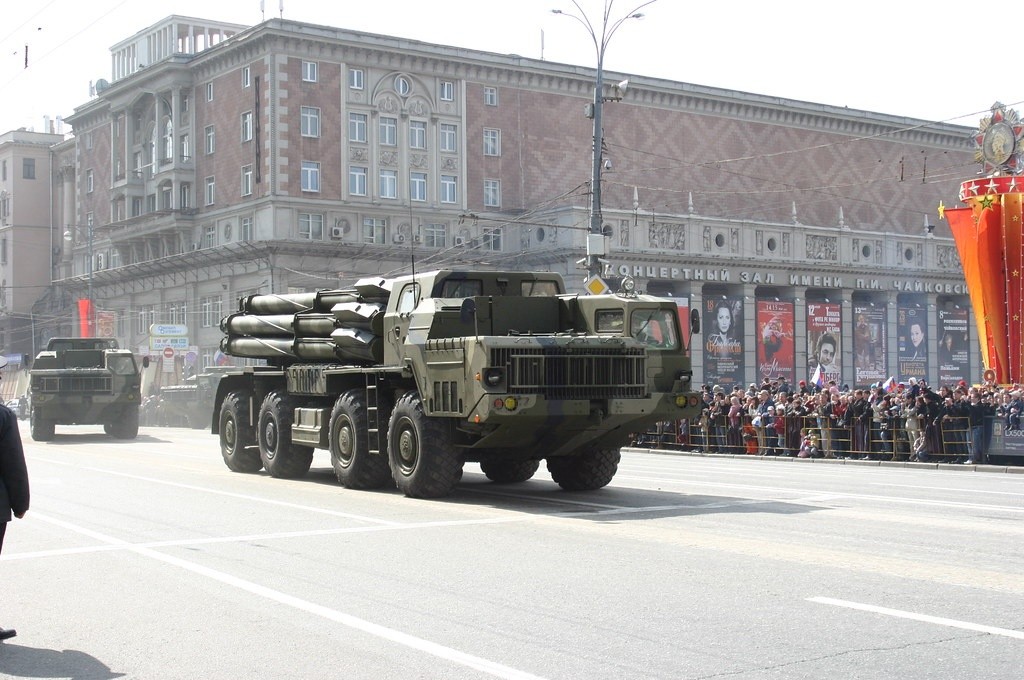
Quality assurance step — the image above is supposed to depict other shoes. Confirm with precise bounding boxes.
[951,459,963,464]
[0,627,16,639]
[938,457,950,463]
[963,459,972,464]
[863,455,879,460]
[1003,425,1010,430]
[1009,426,1015,431]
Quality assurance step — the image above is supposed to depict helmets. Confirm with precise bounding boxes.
[750,383,756,388]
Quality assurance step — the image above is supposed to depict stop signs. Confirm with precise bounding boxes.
[163,348,175,358]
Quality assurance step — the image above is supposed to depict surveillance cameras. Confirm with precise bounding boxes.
[604,160,612,169]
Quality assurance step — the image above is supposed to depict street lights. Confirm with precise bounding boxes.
[550,7,645,297]
[63,225,94,337]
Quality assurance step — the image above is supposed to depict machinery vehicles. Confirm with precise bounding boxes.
[203,266,703,503]
[17,337,152,441]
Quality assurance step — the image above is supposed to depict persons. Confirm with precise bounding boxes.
[854,314,871,371]
[911,320,927,357]
[0,354,30,640]
[807,334,840,385]
[629,377,1023,466]
[707,300,737,358]
[138,394,168,427]
[18,394,30,421]
[938,334,956,366]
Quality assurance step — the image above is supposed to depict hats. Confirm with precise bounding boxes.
[799,381,805,385]
[871,383,876,388]
[960,381,965,386]
[1009,391,1020,397]
[767,405,774,410]
[759,390,768,395]
